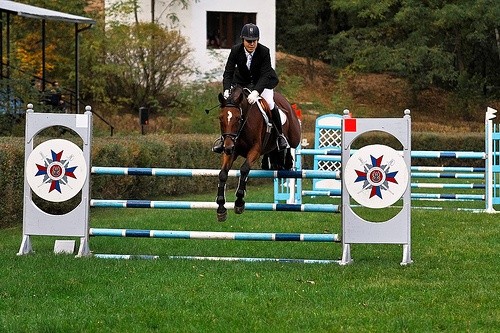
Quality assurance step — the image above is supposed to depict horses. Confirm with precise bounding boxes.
[209,82,302,224]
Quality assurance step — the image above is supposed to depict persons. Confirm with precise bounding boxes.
[211,23,290,153]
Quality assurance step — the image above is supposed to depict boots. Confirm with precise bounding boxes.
[211,141,223,152]
[269,105,287,149]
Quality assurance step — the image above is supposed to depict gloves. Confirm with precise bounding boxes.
[223,90,230,100]
[246,90,259,105]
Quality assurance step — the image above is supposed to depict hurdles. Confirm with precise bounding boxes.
[311,108,500,201]
[273,106,500,217]
[16,105,419,266]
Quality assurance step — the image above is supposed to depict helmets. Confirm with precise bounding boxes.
[240,23,260,41]
